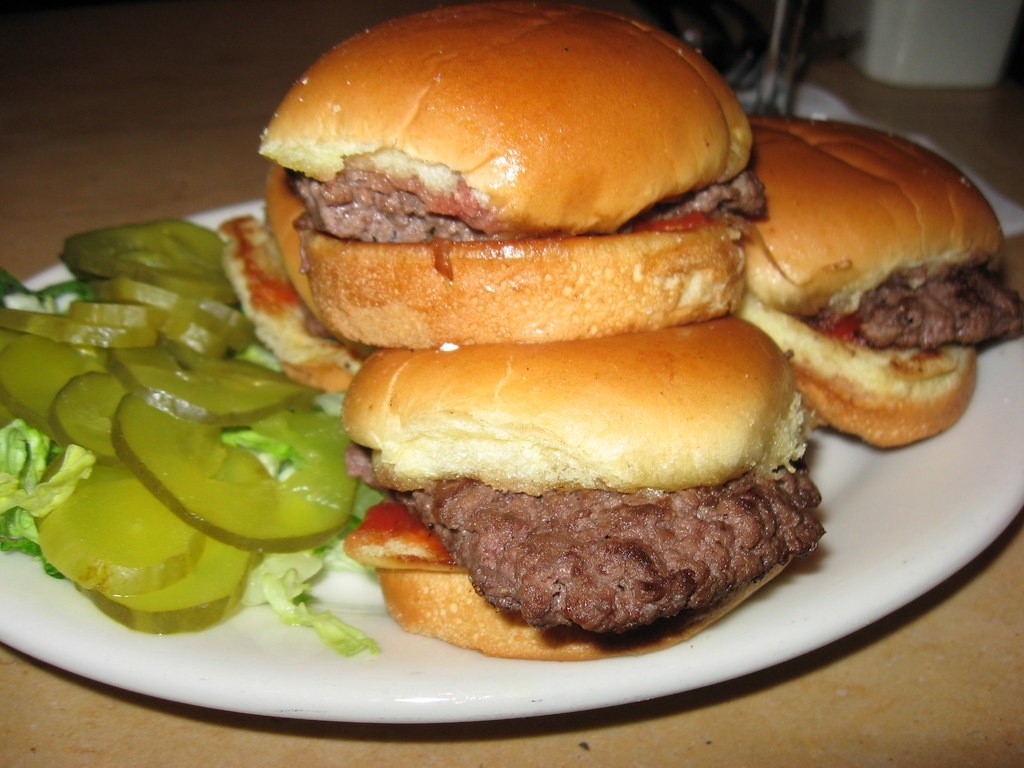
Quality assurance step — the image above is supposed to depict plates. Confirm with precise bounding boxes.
[0,85,1024,723]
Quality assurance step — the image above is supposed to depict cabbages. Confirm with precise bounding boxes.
[0,417,394,656]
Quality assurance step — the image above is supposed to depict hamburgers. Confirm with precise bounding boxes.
[254,4,1024,662]
